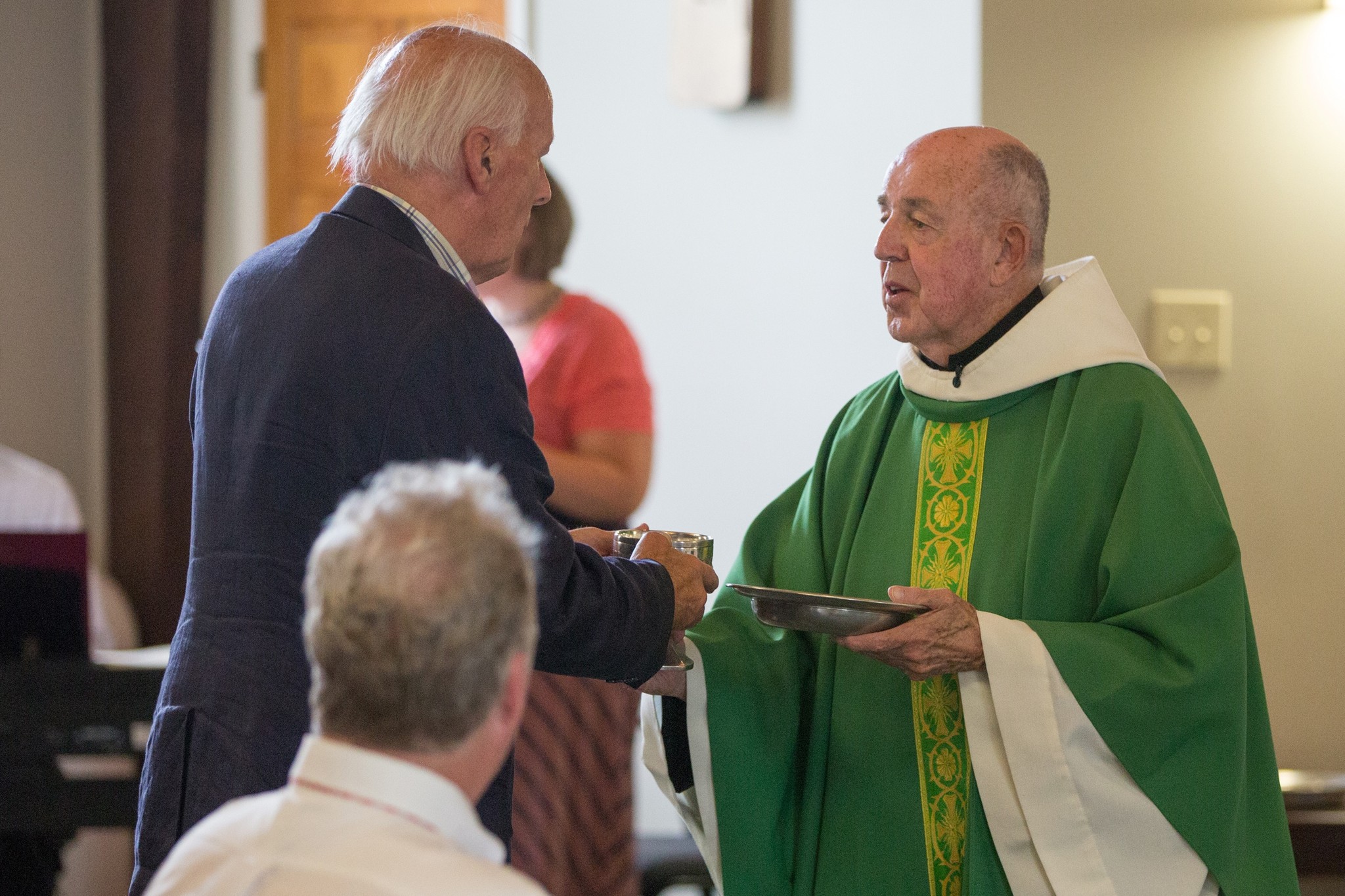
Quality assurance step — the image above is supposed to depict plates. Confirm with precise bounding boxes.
[725,582,931,636]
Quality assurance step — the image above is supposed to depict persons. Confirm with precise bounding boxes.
[474,169,661,896]
[128,24,725,896]
[132,458,560,896]
[604,127,1303,896]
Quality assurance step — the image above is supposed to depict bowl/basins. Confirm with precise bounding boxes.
[611,529,714,569]
